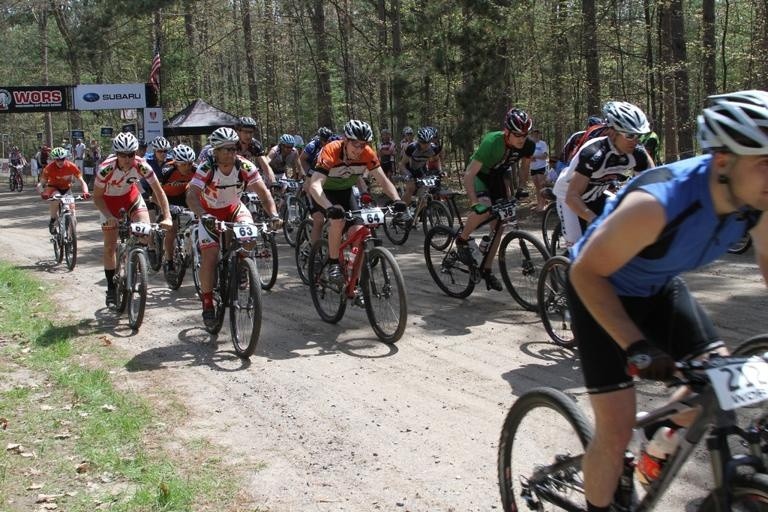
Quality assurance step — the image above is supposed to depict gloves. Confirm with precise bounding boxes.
[404,175,414,184]
[471,203,489,215]
[360,192,372,204]
[83,192,90,200]
[170,204,182,217]
[270,182,281,193]
[41,192,49,200]
[626,339,678,382]
[326,205,346,220]
[201,213,216,229]
[268,214,283,230]
[390,200,406,211]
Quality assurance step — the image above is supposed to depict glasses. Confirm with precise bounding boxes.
[351,140,366,148]
[621,134,643,142]
[179,163,192,167]
[513,133,528,137]
[223,147,240,154]
[56,157,65,162]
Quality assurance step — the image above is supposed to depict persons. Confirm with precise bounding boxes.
[9,138,100,185]
[456,107,665,293]
[93,117,450,328]
[562,88,768,512]
[36,147,91,253]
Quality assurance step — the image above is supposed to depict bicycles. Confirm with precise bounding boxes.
[43,191,90,267]
[306,202,411,345]
[538,191,767,361]
[496,332,767,511]
[143,176,455,291]
[191,216,286,359]
[7,164,26,193]
[423,187,559,314]
[96,209,155,328]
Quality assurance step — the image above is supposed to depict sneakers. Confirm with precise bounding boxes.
[239,267,248,290]
[147,246,157,265]
[402,210,413,221]
[106,287,117,310]
[328,263,346,285]
[163,259,175,276]
[633,410,680,502]
[345,287,366,308]
[480,268,503,290]
[456,238,474,266]
[202,306,217,328]
[49,222,57,236]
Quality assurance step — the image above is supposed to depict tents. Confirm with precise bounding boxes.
[161,99,239,138]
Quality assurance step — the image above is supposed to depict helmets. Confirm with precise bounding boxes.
[343,119,374,143]
[380,129,392,137]
[113,132,196,163]
[209,127,240,149]
[601,100,651,136]
[234,116,258,130]
[279,127,342,148]
[12,146,51,153]
[504,107,532,135]
[50,146,67,160]
[587,116,602,126]
[403,126,438,144]
[694,90,768,156]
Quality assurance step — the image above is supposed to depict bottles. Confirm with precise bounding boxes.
[637,424,679,483]
[479,236,491,254]
[348,246,357,268]
